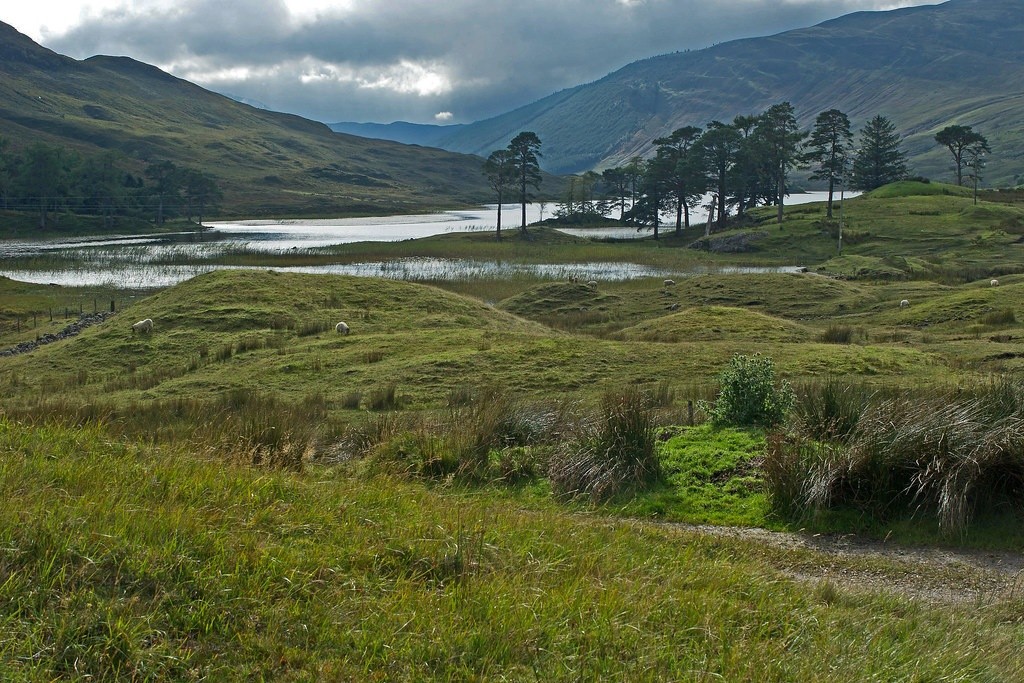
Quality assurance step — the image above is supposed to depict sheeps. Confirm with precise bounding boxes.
[991,279,999,286]
[336,322,350,336]
[587,281,598,290]
[132,319,154,333]
[663,280,675,287]
[900,300,911,309]
